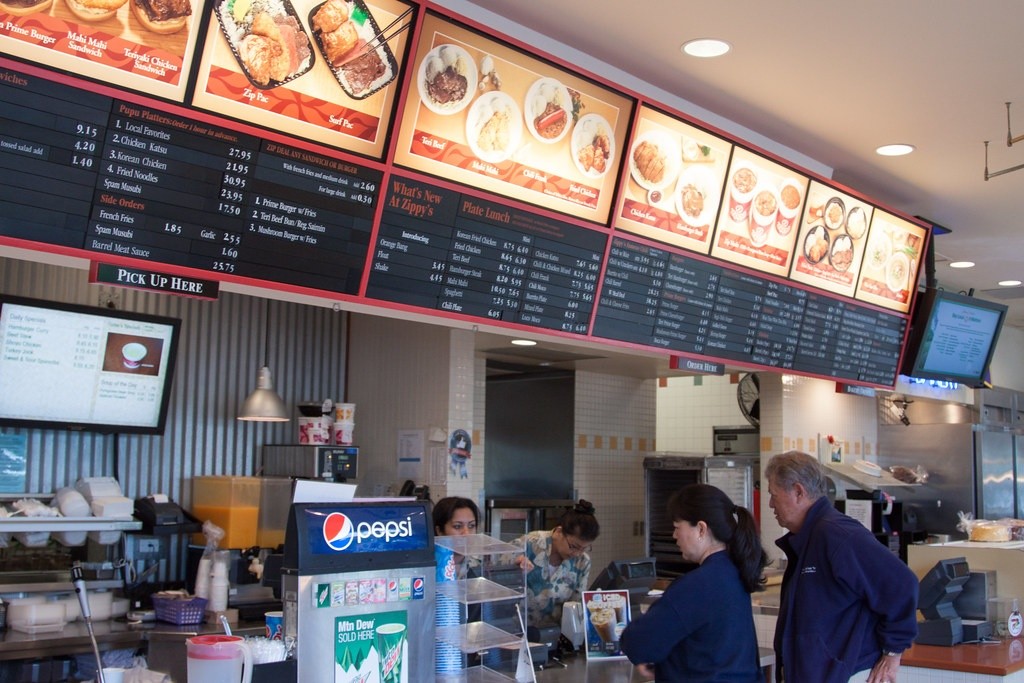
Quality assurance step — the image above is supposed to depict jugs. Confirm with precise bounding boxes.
[186,634,253,683]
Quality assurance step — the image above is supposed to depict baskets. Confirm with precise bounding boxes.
[154,596,207,624]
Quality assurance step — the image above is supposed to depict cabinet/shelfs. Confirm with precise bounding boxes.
[0,493,168,683]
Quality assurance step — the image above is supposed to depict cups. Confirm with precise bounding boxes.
[729,161,760,223]
[749,188,778,246]
[434,538,462,683]
[121,343,147,369]
[298,417,333,445]
[775,179,803,235]
[376,623,406,683]
[988,597,1018,638]
[264,612,283,639]
[333,422,355,445]
[588,596,626,642]
[95,668,126,683]
[195,559,228,612]
[335,403,356,423]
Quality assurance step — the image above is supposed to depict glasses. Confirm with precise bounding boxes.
[563,531,592,554]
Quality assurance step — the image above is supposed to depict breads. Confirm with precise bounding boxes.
[130,0,186,34]
[63,0,128,21]
[0,0,53,16]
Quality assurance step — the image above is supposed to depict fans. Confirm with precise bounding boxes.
[879,392,913,426]
[737,372,760,428]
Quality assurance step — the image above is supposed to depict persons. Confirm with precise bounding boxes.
[496,500,599,626]
[766,450,918,683]
[433,496,481,625]
[620,482,768,683]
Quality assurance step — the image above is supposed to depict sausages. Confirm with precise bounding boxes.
[538,109,565,129]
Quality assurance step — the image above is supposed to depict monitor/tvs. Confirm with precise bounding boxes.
[0,293,183,436]
[901,288,1009,387]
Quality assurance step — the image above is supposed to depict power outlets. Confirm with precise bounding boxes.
[140,539,159,552]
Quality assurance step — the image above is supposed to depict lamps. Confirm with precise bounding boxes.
[237,299,291,422]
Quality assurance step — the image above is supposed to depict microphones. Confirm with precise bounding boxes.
[69,565,105,683]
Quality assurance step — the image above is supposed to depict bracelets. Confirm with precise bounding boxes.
[882,650,900,656]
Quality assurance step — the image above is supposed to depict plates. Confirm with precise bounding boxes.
[845,206,867,239]
[466,90,523,164]
[803,224,830,264]
[417,44,479,115]
[674,164,720,226]
[629,128,682,189]
[885,252,909,293]
[867,230,893,270]
[6,591,130,633]
[523,77,574,143]
[571,113,616,178]
[824,196,845,230]
[829,233,854,272]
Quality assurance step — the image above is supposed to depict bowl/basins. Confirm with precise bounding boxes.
[213,0,316,89]
[297,401,335,418]
[308,0,399,99]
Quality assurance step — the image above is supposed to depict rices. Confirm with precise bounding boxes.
[217,0,310,78]
[326,1,392,98]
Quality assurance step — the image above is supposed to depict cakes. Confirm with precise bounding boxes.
[969,525,1024,542]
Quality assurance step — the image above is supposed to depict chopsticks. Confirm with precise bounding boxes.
[360,6,414,60]
